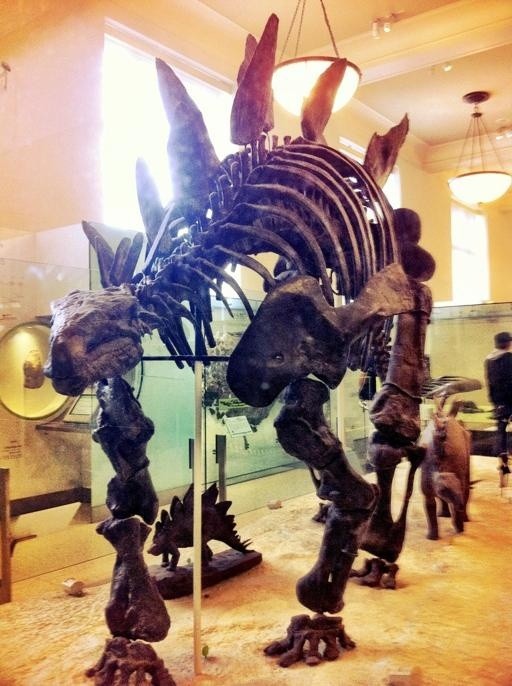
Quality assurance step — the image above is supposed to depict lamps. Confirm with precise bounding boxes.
[270,1,362,120]
[371,10,395,39]
[448,90,512,205]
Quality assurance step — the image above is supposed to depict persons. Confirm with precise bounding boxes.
[482,330,511,475]
